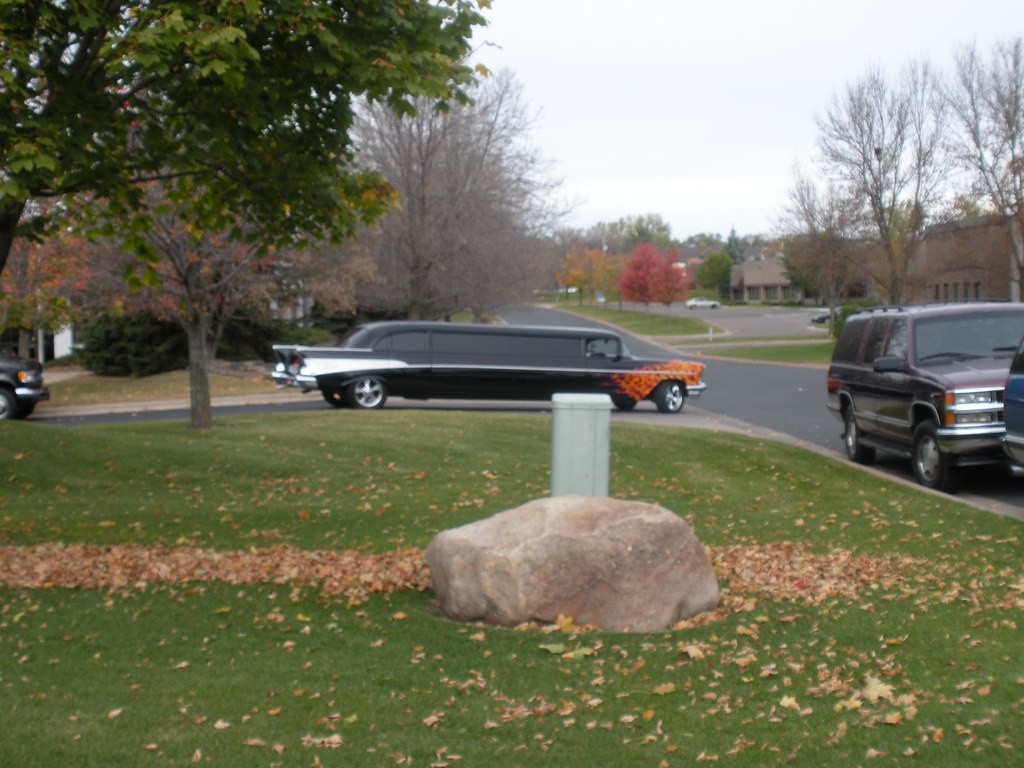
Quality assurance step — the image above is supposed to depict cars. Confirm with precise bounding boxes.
[268,319,707,411]
[1,351,49,421]
[685,296,720,310]
[811,305,874,324]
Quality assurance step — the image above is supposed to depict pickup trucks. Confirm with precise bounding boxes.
[1000,336,1024,477]
[826,300,1024,493]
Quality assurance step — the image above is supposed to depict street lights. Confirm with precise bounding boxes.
[874,146,884,208]
